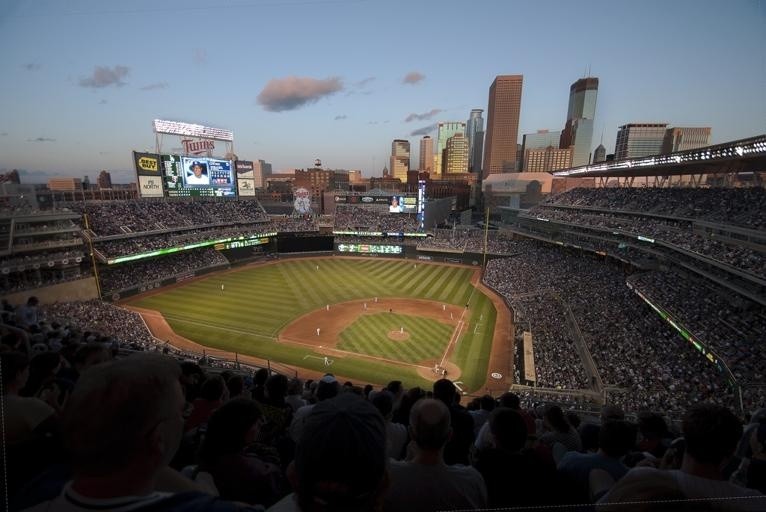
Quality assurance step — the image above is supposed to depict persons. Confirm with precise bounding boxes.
[390,196,402,214]
[484,186,766,298]
[186,160,209,184]
[0,195,276,296]
[275,207,489,255]
[1,298,764,511]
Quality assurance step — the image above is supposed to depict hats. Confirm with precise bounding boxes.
[293,386,393,504]
